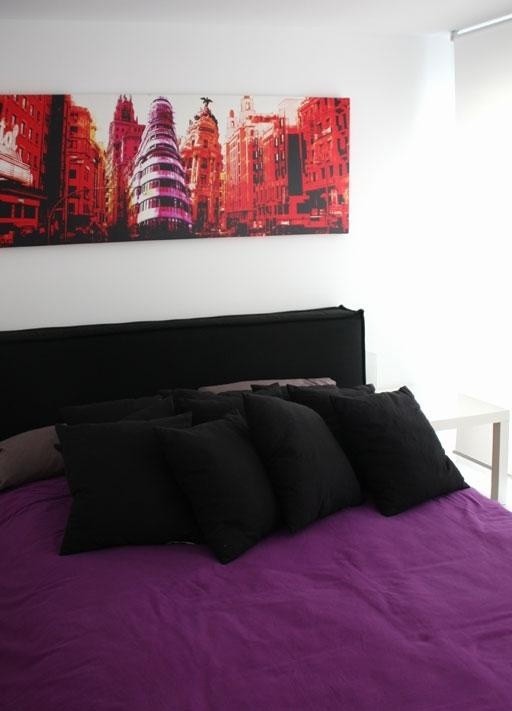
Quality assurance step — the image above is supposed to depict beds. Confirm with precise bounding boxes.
[1,306,512,710]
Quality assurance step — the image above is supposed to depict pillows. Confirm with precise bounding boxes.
[332,386,467,517]
[135,410,277,561]
[252,382,375,407]
[169,383,288,424]
[51,416,192,553]
[51,392,171,417]
[288,383,369,423]
[240,394,364,536]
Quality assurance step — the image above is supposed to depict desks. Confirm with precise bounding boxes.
[412,390,510,505]
